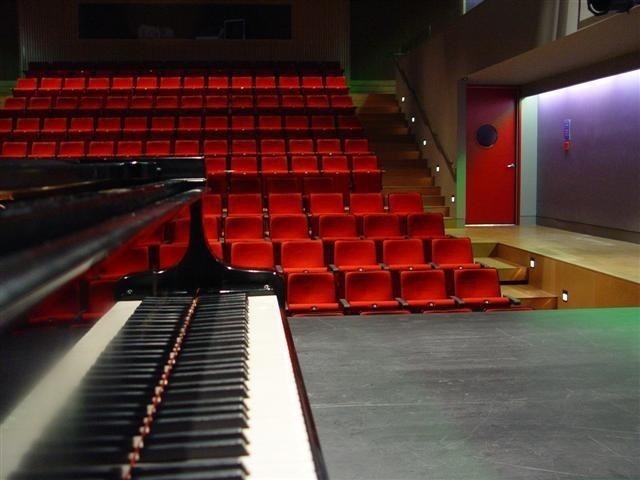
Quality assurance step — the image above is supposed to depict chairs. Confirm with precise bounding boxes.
[201,193,533,317]
[0,61,383,195]
[87,200,191,298]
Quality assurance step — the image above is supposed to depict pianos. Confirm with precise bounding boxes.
[1,155,327,480]
